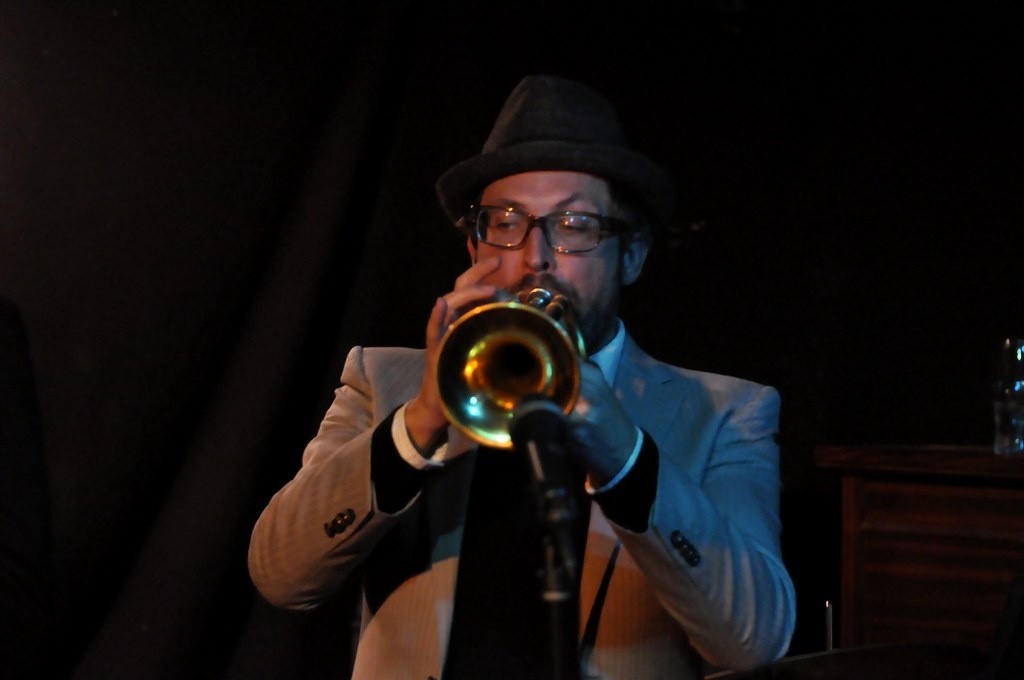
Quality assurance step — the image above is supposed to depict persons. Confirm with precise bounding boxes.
[247,78,798,680]
[0,294,55,680]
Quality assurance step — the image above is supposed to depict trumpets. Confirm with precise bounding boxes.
[427,289,588,452]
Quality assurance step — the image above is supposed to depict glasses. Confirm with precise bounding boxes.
[464,202,632,254]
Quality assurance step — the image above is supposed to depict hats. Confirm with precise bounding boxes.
[433,75,675,230]
